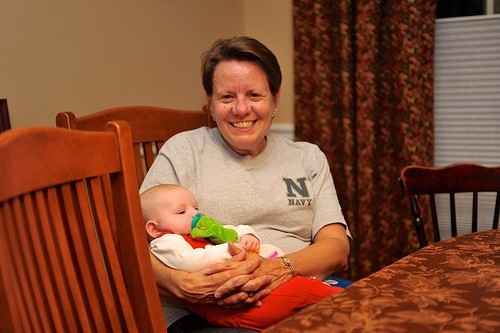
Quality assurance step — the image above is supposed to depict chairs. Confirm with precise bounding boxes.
[401,162,499,246]
[56,106,219,188]
[0,121,166,333]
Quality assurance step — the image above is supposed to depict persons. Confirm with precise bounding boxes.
[138,35,353,333]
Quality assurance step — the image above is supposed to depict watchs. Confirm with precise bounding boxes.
[277,256,296,276]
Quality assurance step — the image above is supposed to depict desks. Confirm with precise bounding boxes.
[259,229,500,333]
